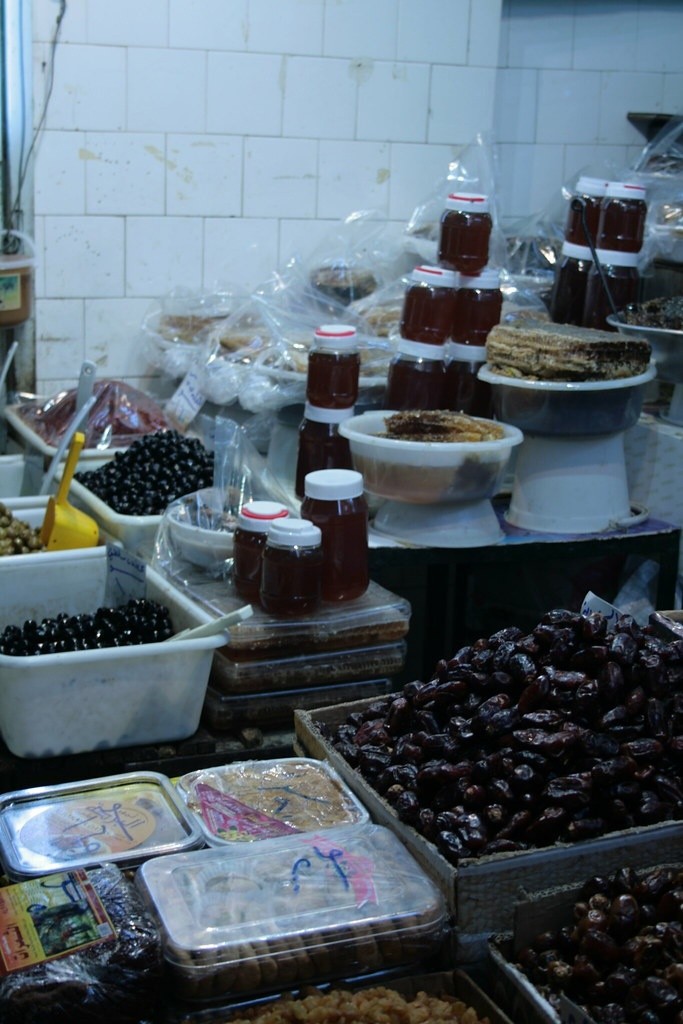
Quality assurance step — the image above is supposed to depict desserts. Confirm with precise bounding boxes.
[360,319,653,444]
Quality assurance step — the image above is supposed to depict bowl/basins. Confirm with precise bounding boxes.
[606,309,683,384]
[659,385,683,426]
[476,358,656,437]
[166,488,233,570]
[338,410,522,499]
[368,498,506,547]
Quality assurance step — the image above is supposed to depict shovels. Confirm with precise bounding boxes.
[161,602,256,640]
[39,431,101,548]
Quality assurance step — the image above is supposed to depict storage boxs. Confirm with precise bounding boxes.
[0,369,683,1023]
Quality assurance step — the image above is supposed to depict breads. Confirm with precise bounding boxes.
[163,269,413,375]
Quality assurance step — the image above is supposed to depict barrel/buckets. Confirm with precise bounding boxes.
[502,432,651,535]
